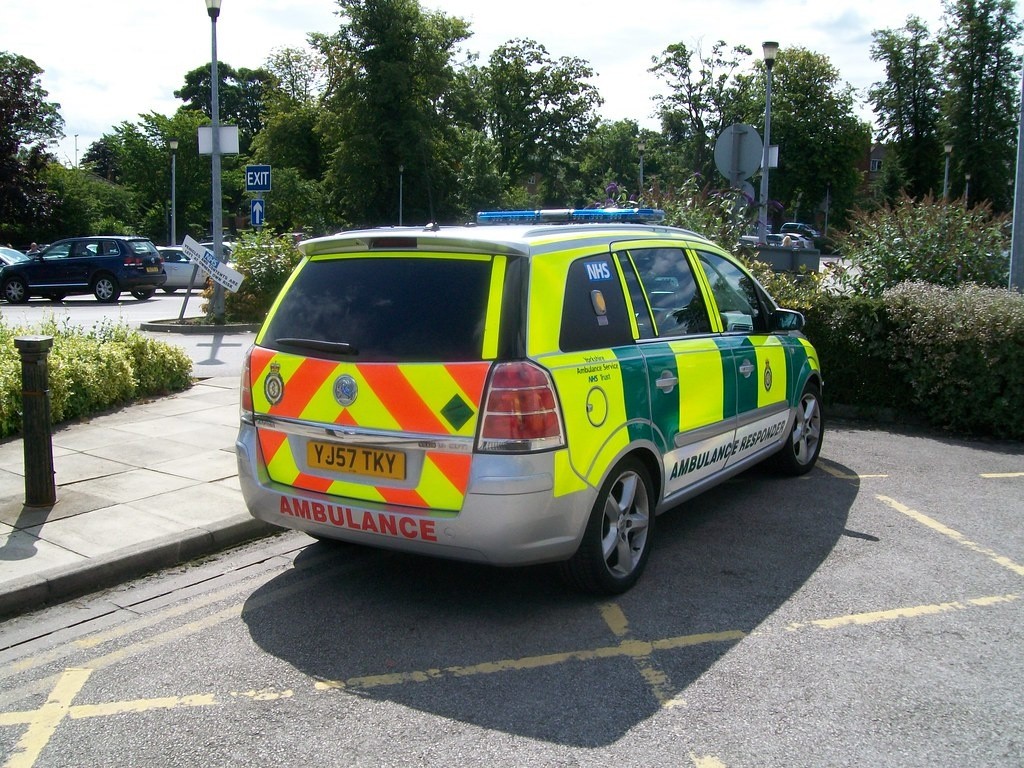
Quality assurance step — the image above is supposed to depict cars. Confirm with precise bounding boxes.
[199,231,304,271]
[155,245,243,294]
[35,242,111,258]
[0,246,31,269]
[781,223,821,240]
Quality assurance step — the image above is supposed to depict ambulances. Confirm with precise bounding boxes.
[233,207,829,589]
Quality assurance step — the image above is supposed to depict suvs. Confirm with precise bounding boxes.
[0,236,168,303]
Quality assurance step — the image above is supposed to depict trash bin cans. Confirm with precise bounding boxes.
[738,239,821,284]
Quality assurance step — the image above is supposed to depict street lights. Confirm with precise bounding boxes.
[637,138,646,202]
[755,39,779,242]
[962,171,971,212]
[942,140,955,203]
[168,139,180,245]
[399,164,405,227]
[205,0,226,319]
